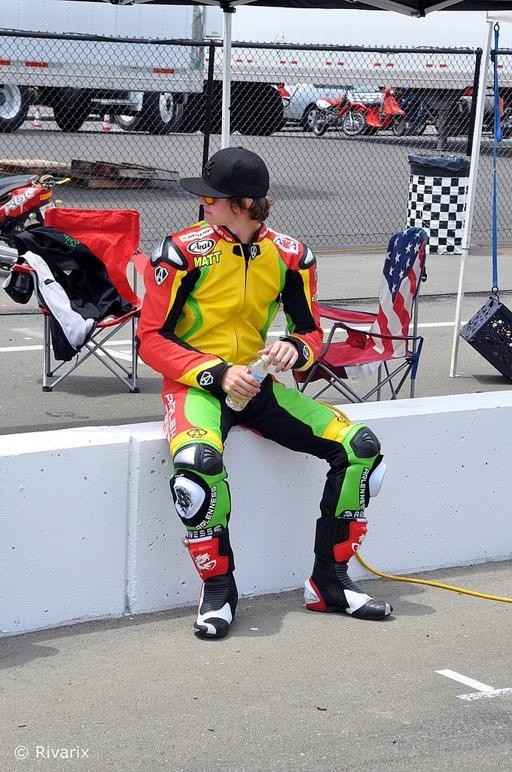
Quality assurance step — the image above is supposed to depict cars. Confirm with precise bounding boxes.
[270,83,385,131]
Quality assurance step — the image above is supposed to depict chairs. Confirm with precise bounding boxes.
[39,206,150,393]
[293,227,431,401]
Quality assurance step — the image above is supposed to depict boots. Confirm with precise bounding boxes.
[181,526,242,640]
[302,513,394,620]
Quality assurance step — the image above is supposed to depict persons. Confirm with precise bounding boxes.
[135,144,394,640]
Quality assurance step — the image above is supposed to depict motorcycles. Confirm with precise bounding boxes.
[312,85,426,136]
[0,173,71,273]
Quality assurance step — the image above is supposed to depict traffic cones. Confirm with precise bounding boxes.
[33,106,43,129]
[102,114,112,132]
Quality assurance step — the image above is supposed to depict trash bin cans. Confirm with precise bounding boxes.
[404,152,471,255]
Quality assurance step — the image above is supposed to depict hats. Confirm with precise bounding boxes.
[179,145,270,199]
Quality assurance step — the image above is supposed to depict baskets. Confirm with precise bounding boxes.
[459,295,512,380]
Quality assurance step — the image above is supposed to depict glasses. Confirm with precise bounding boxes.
[204,196,230,206]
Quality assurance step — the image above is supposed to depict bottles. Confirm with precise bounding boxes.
[225,354,273,411]
